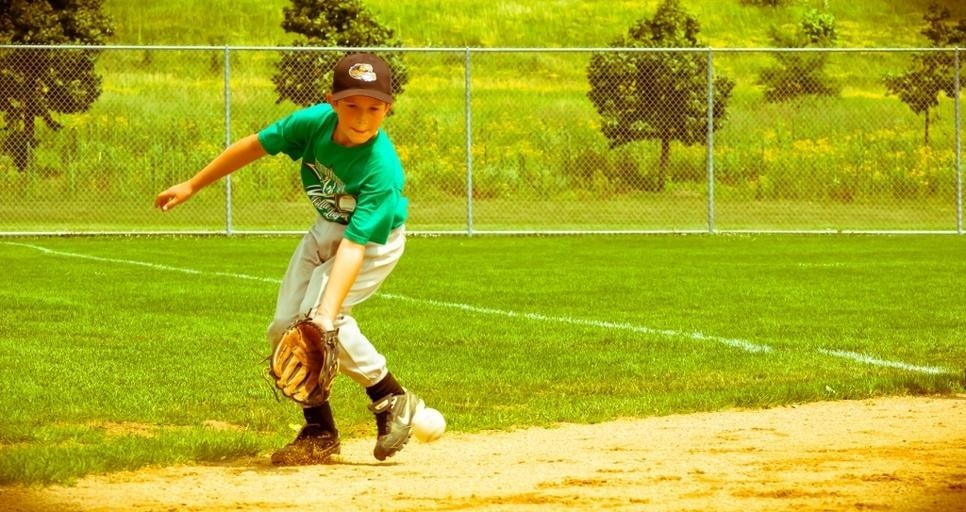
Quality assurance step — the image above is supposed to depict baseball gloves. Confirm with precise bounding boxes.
[270,319,340,406]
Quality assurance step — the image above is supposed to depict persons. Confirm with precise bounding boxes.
[153,50,417,468]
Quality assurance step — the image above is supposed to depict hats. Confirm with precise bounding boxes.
[332,53,394,104]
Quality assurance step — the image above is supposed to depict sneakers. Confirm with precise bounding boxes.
[270,422,341,466]
[366,386,426,461]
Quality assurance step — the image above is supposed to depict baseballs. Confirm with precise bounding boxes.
[412,409,446,443]
